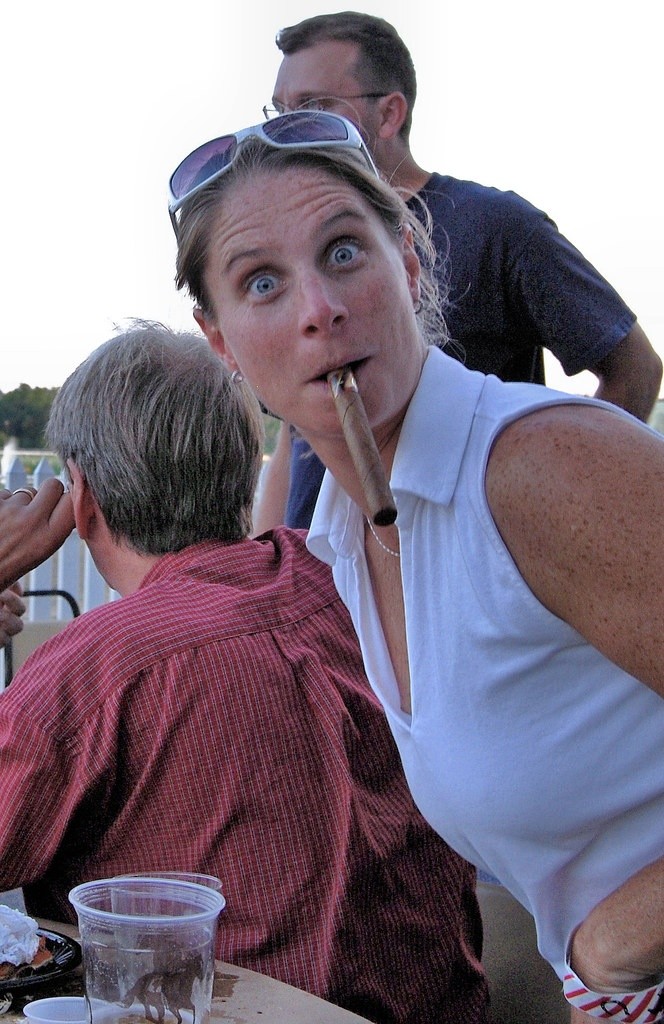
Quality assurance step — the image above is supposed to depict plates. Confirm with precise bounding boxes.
[0,926,83,993]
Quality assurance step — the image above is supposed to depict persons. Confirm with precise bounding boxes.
[250,11,664,543]
[1,478,75,650]
[1,327,497,1024]
[166,109,663,1024]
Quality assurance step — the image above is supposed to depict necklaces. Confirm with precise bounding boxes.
[366,516,400,556]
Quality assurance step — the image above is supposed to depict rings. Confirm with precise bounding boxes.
[12,488,34,500]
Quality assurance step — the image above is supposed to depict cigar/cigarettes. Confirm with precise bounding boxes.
[326,365,402,527]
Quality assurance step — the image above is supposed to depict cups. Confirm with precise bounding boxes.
[67,871,227,1019]
[23,998,107,1024]
[88,1000,201,1024]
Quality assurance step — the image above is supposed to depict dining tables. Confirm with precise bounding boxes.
[0,916,374,1022]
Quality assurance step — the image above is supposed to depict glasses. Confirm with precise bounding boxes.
[167,110,379,239]
[263,92,392,127]
